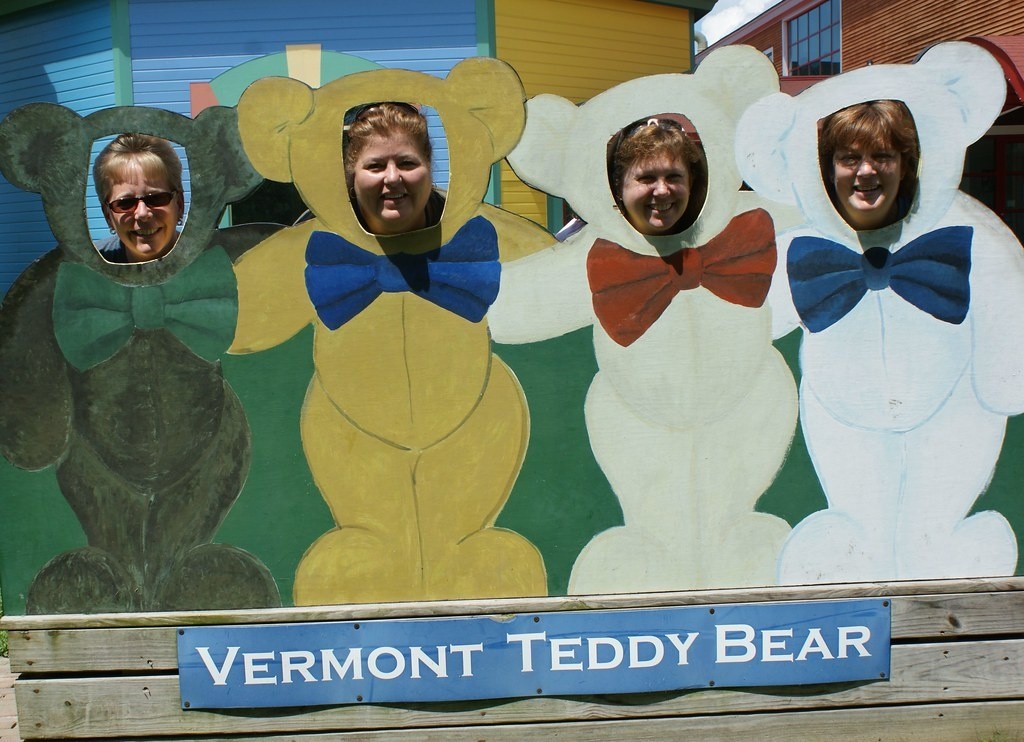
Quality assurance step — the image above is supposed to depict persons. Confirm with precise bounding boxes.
[607,118,708,237]
[818,100,919,229]
[94,134,185,264]
[343,105,445,235]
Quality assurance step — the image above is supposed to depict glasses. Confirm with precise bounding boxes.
[354,101,420,122]
[106,191,174,213]
[615,117,688,159]
